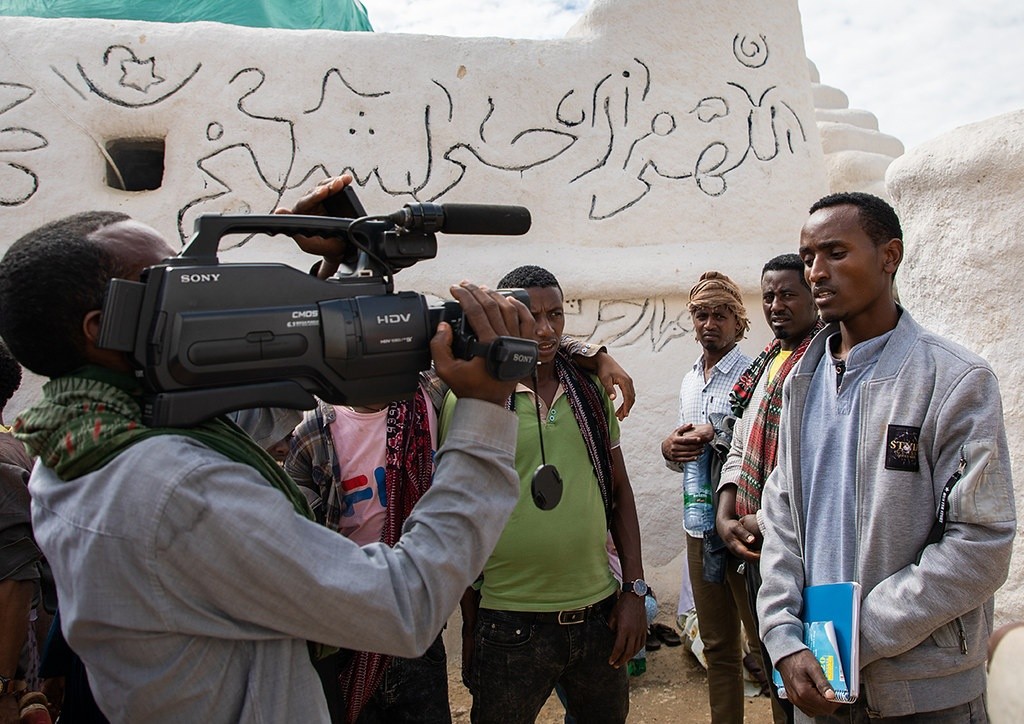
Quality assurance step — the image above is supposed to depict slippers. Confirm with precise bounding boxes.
[652,623,682,647]
[646,627,662,651]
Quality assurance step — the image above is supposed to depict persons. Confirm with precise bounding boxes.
[0,252,647,724]
[0,173,536,724]
[715,254,845,724]
[756,192,1016,724]
[661,272,789,724]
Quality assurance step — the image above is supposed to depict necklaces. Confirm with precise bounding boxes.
[363,406,387,412]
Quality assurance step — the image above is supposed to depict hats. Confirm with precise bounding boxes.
[689,270,751,343]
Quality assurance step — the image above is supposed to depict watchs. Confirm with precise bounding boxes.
[622,579,651,597]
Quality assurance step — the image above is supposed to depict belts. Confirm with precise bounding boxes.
[490,595,617,625]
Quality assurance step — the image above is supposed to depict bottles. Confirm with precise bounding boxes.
[627,645,646,685]
[683,443,715,538]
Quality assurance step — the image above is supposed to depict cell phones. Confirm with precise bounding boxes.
[297,187,366,238]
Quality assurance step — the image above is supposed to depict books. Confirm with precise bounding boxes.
[772,582,862,703]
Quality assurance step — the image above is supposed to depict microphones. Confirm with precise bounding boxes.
[389,203,530,235]
